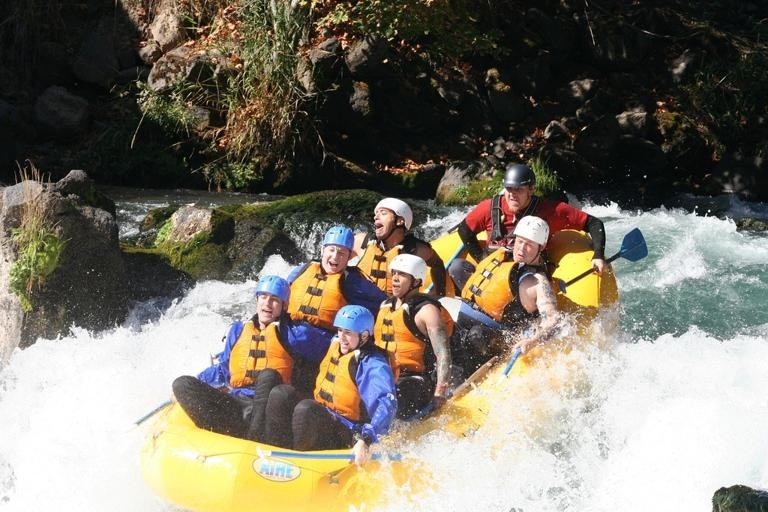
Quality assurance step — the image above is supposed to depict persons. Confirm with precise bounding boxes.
[286,225,389,334]
[171,274,291,443]
[266,304,399,467]
[374,254,455,422]
[348,197,446,298]
[437,214,559,382]
[447,163,609,292]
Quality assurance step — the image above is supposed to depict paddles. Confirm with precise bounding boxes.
[558,228,648,294]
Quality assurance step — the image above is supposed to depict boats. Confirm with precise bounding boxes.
[137,225,618,512]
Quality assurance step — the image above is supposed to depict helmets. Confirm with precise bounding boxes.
[504,164,537,189]
[333,304,375,336]
[256,275,291,302]
[323,224,354,251]
[389,252,427,285]
[374,196,413,231]
[514,216,550,247]
